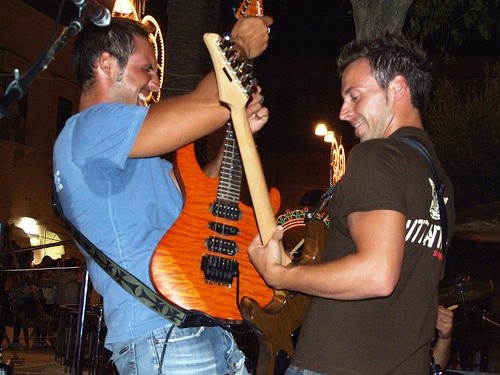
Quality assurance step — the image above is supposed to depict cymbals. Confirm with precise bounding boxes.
[453,202,500,242]
[439,272,500,305]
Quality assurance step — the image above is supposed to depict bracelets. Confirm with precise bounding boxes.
[438,331,452,338]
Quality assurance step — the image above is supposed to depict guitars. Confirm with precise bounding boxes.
[202,31,328,375]
[149,0,282,325]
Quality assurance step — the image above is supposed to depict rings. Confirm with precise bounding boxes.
[268,27,270,33]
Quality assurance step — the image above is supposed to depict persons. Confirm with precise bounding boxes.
[247,27,456,375]
[51,17,273,375]
[433,303,458,370]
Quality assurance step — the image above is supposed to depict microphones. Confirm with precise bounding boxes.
[73,0,110,26]
